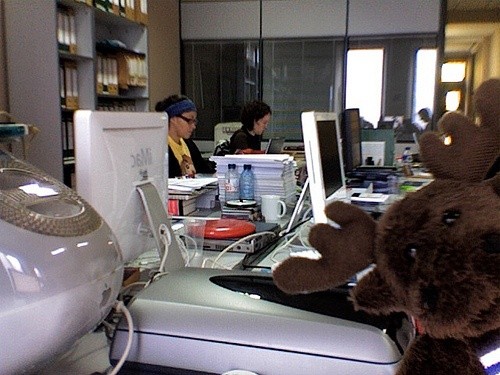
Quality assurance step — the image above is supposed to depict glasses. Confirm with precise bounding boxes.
[177,115,198,125]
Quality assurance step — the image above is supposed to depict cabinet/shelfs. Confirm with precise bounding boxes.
[3,0,151,190]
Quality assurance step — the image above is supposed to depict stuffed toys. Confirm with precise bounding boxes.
[272,77,500,375]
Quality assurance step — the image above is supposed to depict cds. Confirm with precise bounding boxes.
[226,199,257,208]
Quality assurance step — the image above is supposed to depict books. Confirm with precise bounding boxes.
[168,154,297,216]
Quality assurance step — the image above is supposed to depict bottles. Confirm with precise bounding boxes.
[239,164,255,201]
[224,164,240,202]
[403,146,412,169]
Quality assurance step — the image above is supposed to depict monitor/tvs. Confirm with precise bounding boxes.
[341,108,367,179]
[73,109,185,272]
[300,110,384,230]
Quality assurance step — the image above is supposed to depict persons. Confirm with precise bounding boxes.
[155,94,217,179]
[230,100,275,155]
[418,108,436,132]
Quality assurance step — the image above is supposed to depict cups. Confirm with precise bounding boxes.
[386,175,398,194]
[260,194,287,221]
[182,217,207,257]
